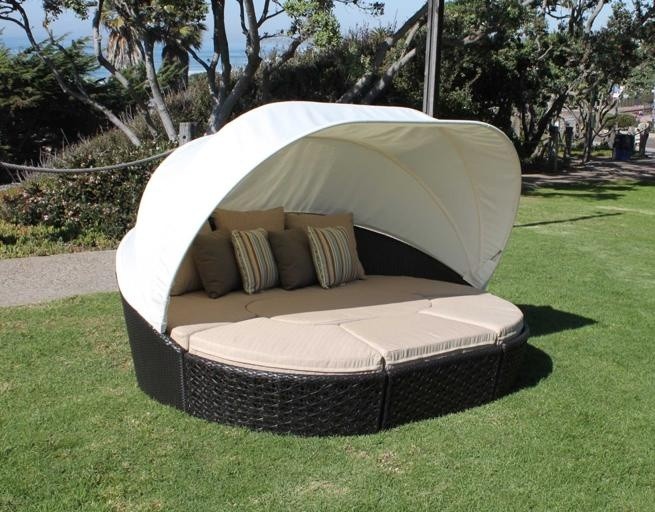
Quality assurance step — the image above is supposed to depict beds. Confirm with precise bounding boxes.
[113,213,530,439]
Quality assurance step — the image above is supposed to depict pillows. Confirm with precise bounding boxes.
[172,206,367,299]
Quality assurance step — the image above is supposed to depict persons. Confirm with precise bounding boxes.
[574,124,580,138]
[561,122,573,154]
[551,120,560,154]
